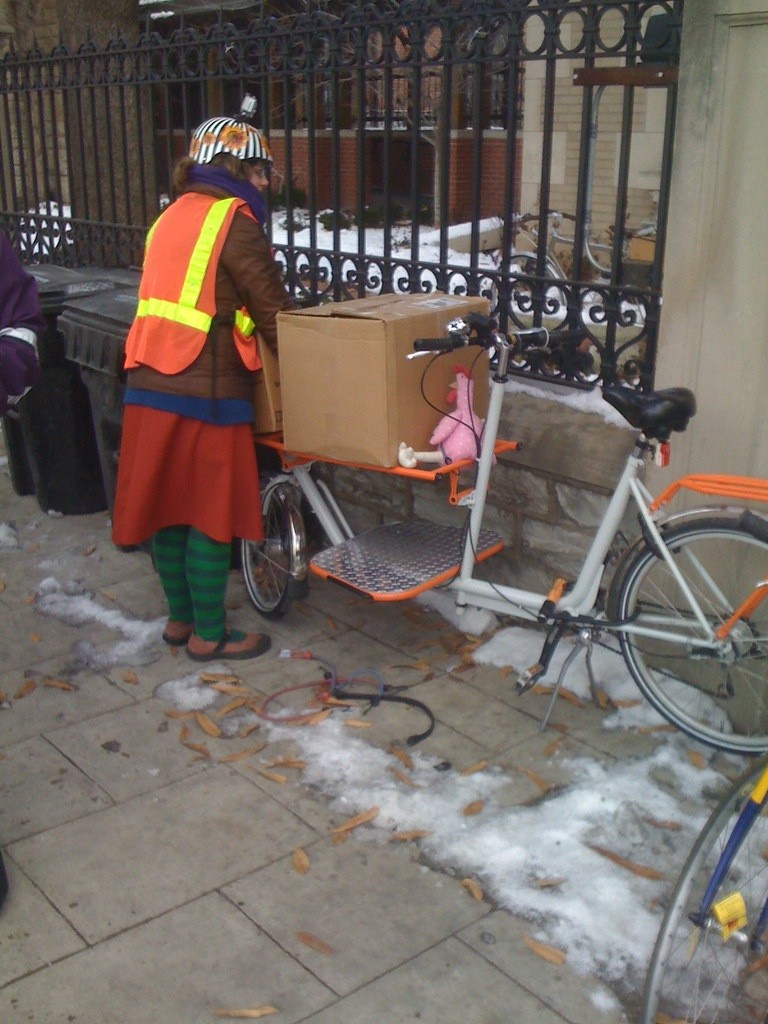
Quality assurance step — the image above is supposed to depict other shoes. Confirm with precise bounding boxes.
[186,624,269,663]
[161,618,196,648]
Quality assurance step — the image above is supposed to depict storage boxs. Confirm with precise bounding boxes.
[629,235,656,261]
[275,292,492,467]
[250,328,283,435]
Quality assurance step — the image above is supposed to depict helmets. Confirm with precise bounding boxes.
[187,116,275,166]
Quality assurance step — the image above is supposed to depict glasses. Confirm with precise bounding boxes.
[251,159,268,178]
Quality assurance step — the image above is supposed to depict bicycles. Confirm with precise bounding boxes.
[642,750,767,1024]
[239,332,768,765]
[498,211,656,332]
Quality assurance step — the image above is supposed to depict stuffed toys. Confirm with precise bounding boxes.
[398,364,497,469]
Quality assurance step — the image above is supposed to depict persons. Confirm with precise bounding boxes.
[112,117,304,662]
[0,232,48,417]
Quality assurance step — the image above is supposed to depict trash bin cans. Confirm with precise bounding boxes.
[7,263,145,517]
[55,285,331,572]
